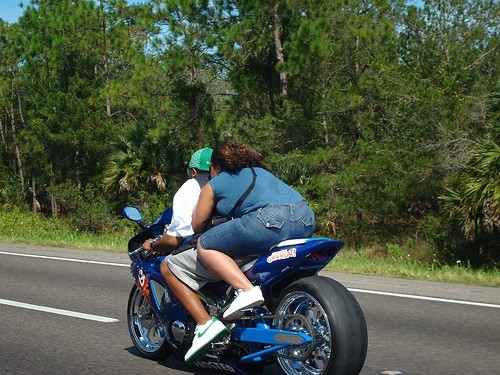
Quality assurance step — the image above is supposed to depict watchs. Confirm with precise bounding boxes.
[149,237,159,253]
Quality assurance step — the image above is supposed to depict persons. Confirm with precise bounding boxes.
[141,146,260,364]
[190,138,316,322]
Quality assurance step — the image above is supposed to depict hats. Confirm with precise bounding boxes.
[188,147,214,171]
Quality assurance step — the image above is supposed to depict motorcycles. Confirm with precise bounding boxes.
[124,205,370,375]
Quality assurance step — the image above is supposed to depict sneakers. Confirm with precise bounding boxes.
[184,315,229,365]
[223,286,265,322]
